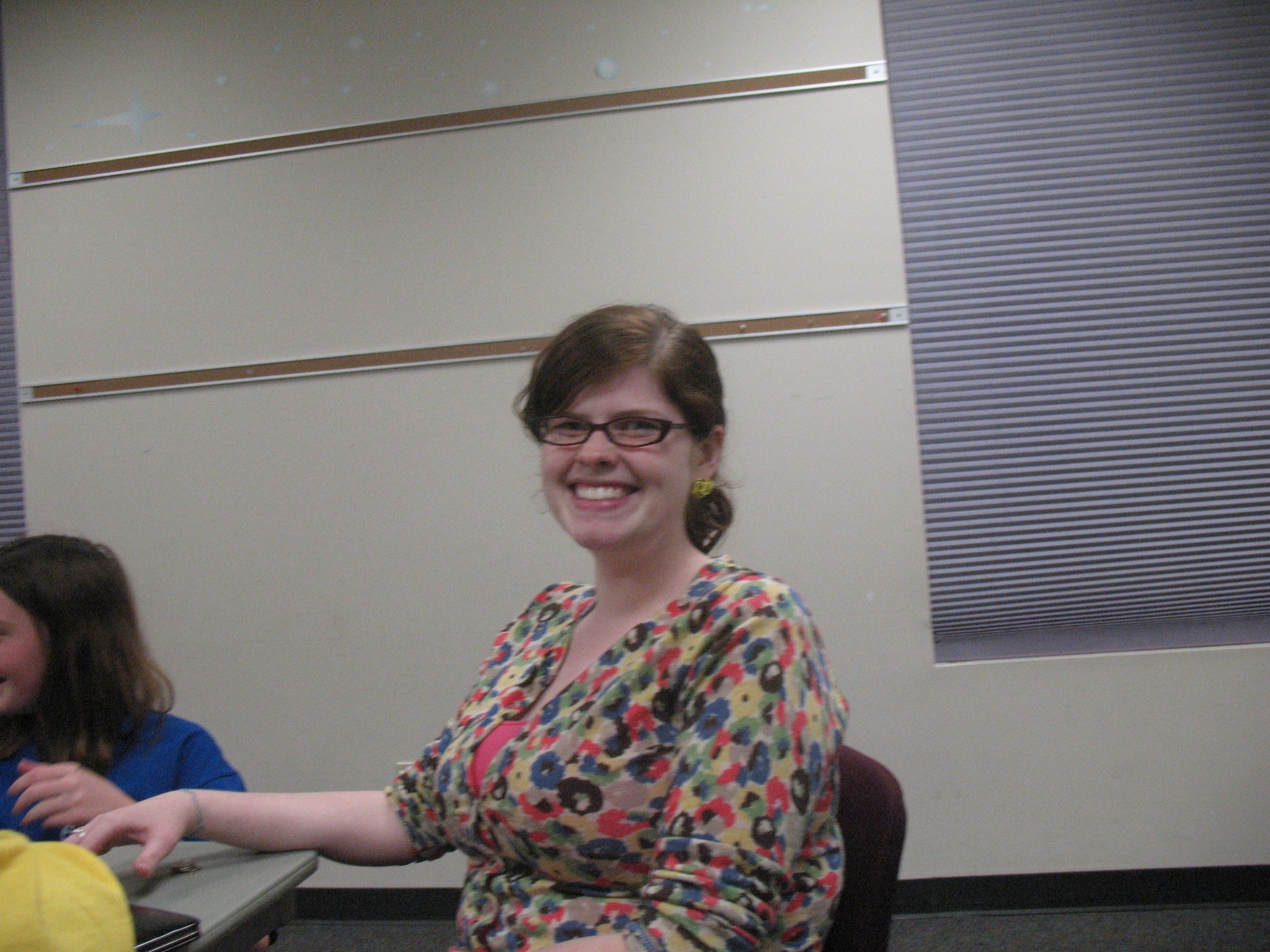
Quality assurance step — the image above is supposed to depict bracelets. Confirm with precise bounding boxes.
[180,787,203,841]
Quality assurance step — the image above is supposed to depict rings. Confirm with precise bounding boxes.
[72,826,86,839]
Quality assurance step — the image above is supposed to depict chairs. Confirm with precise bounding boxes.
[819,741,907,952]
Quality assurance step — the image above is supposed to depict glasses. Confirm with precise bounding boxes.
[533,414,693,449]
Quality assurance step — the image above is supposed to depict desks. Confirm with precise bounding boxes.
[98,838,319,951]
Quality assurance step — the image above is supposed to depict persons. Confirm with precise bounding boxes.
[61,301,854,952]
[0,535,247,842]
